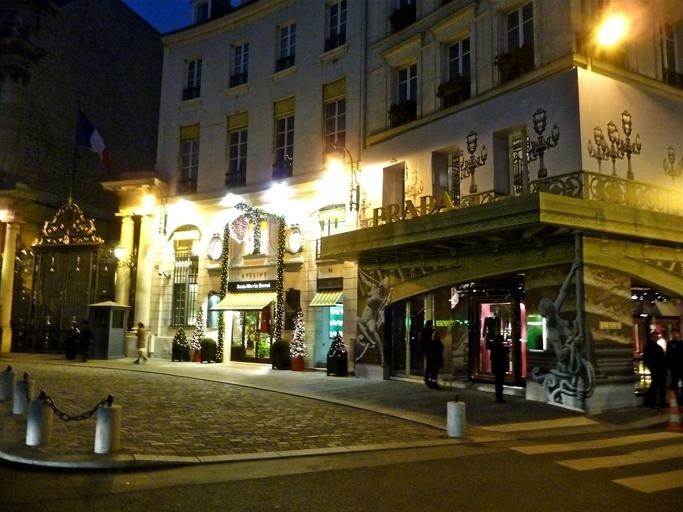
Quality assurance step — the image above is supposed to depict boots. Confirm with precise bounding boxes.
[496,387,506,403]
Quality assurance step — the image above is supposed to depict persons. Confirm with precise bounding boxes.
[488,335,510,403]
[132,321,148,364]
[77,320,95,362]
[417,320,444,390]
[481,312,500,351]
[665,330,683,408]
[641,332,671,410]
[656,334,667,358]
[357,268,391,367]
[63,321,80,360]
[538,261,589,375]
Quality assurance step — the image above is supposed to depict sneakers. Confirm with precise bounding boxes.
[134,357,147,364]
[644,399,670,409]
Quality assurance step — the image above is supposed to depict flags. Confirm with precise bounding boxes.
[75,109,112,175]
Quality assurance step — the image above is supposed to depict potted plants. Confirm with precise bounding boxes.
[172,326,189,362]
[326,329,350,375]
